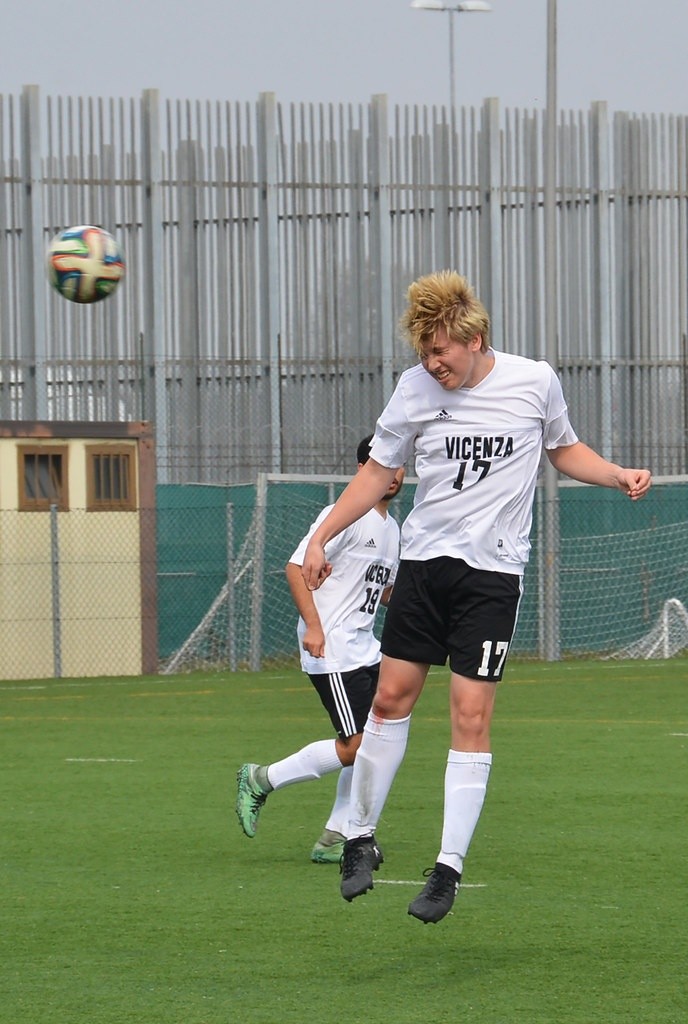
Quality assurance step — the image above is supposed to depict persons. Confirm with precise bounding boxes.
[235,433,407,863]
[301,269,652,925]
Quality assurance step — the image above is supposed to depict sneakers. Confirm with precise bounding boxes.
[408,862,461,924]
[235,764,268,838]
[311,839,346,863]
[339,834,385,903]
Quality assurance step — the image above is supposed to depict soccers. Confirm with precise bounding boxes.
[44,226,124,304]
